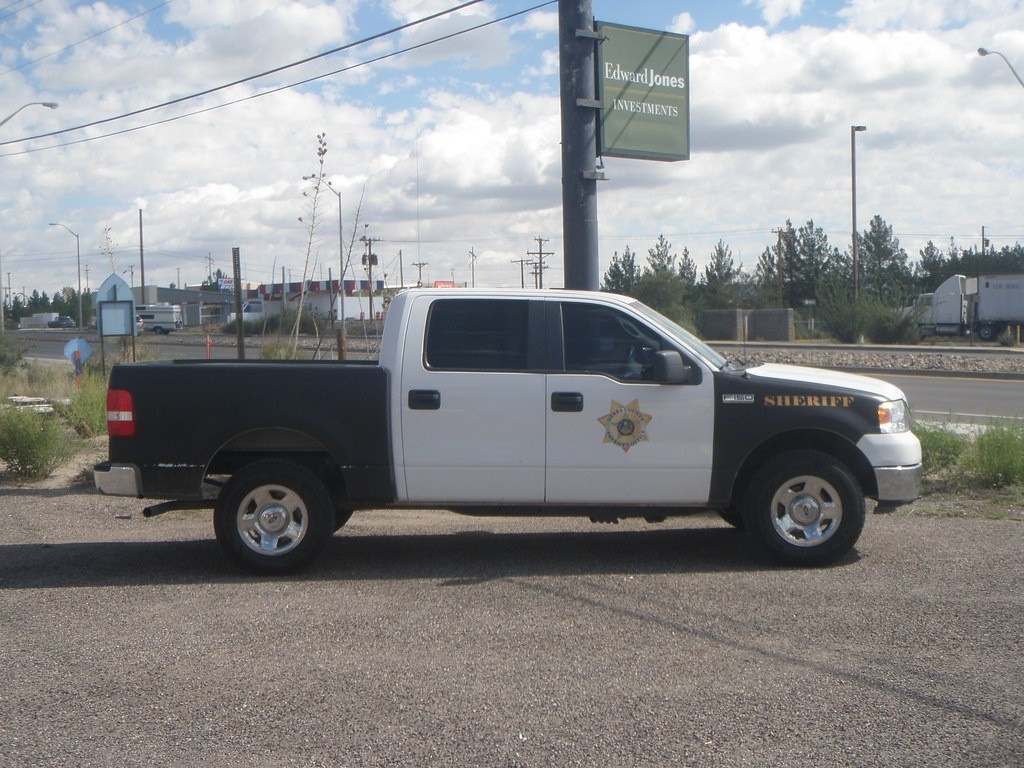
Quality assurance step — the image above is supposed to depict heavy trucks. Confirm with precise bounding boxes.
[227,298,296,326]
[896,274,1024,343]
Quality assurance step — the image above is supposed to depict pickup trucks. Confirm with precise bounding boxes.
[94,286,923,577]
[48,316,76,329]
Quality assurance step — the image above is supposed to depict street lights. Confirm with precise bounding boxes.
[49,222,82,331]
[303,176,345,328]
[851,124,867,300]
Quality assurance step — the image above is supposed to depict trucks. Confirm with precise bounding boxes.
[136,305,183,335]
